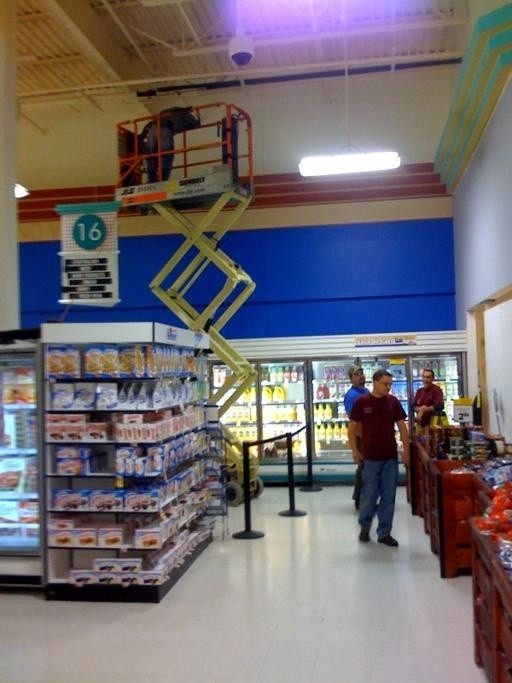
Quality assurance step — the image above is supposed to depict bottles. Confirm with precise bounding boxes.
[412,360,459,378]
[313,402,348,451]
[214,365,306,384]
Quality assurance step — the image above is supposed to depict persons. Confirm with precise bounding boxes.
[411,369,444,428]
[348,370,411,547]
[344,364,379,511]
[140,106,199,184]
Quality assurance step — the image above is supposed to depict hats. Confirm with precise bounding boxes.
[348,363,363,376]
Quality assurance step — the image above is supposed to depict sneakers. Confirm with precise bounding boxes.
[360,529,371,542]
[376,535,399,546]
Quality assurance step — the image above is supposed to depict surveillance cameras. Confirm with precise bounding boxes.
[228,36,255,67]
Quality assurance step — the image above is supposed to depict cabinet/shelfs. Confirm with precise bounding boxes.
[0,322,226,603]
